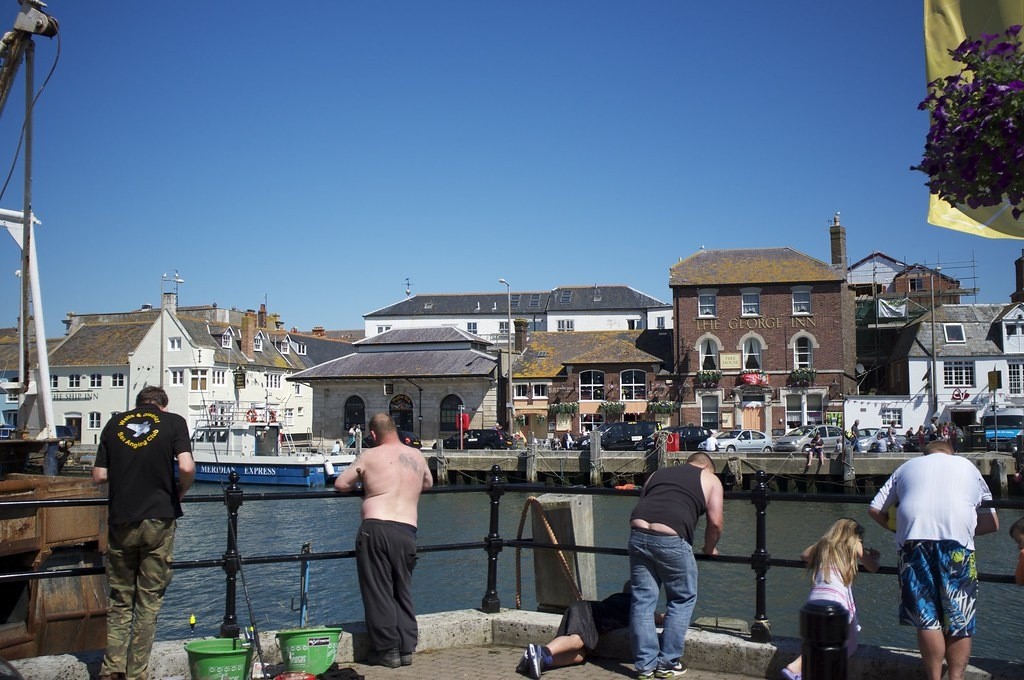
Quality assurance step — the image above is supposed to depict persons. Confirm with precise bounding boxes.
[872,434,887,453]
[906,418,958,452]
[493,423,501,430]
[868,441,999,680]
[518,581,664,680]
[705,433,719,451]
[807,434,824,466]
[888,421,897,450]
[92,386,196,680]
[781,518,881,680]
[1009,517,1024,585]
[331,441,341,455]
[834,439,842,453]
[852,420,861,453]
[335,413,433,667]
[346,424,360,448]
[628,452,724,680]
[553,429,575,450]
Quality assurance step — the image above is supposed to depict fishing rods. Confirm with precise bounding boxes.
[356,468,363,482]
[191,346,267,680]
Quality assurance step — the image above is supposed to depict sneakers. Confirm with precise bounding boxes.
[637,671,655,680]
[528,644,543,679]
[517,650,547,671]
[655,661,690,678]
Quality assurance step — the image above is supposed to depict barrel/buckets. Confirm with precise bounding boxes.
[275,627,344,676]
[184,638,252,680]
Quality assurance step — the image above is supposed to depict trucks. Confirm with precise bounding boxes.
[981,403,1024,440]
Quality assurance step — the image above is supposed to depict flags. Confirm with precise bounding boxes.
[924,0,1024,239]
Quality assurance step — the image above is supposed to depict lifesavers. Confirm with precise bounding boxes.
[208,404,218,413]
[246,410,257,422]
[263,411,276,422]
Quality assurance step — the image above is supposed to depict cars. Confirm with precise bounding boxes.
[633,426,713,451]
[0,424,15,440]
[774,425,850,452]
[55,425,75,447]
[911,424,964,450]
[432,429,517,450]
[698,428,774,452]
[844,427,906,453]
[362,430,422,450]
[572,420,662,451]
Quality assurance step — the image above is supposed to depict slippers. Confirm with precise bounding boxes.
[781,668,802,680]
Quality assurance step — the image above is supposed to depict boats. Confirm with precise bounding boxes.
[172,399,360,486]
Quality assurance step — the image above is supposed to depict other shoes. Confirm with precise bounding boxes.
[99,672,126,680]
[401,652,412,666]
[366,647,401,668]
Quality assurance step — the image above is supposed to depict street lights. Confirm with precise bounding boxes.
[159,278,184,389]
[930,267,942,404]
[458,405,465,450]
[499,279,513,435]
